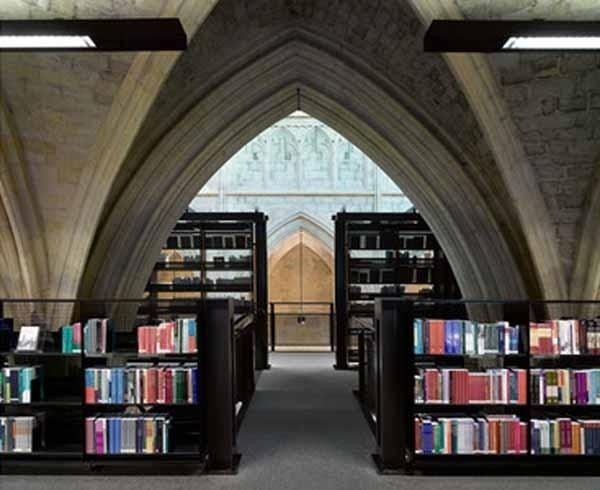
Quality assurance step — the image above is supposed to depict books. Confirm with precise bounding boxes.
[0,315,202,458]
[347,227,448,314]
[413,315,600,458]
[162,233,254,308]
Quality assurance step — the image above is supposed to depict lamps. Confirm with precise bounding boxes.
[0,16,188,53]
[424,20,600,54]
